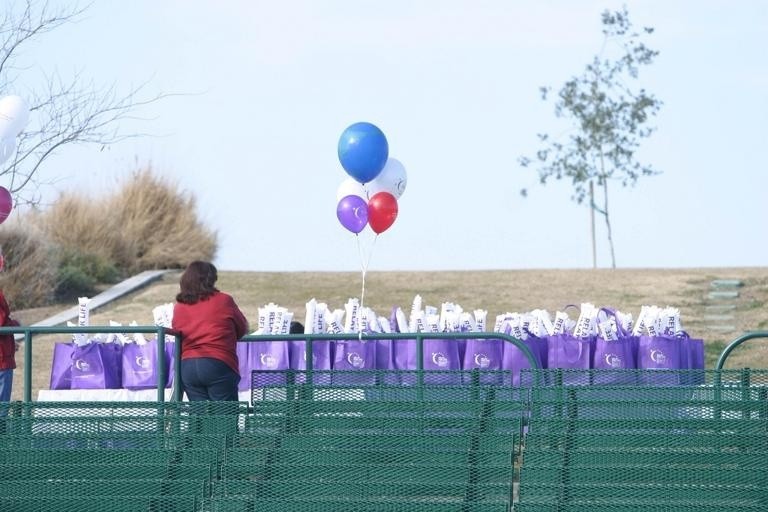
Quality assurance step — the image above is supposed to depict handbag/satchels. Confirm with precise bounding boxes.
[235,306,705,391]
[50,321,176,390]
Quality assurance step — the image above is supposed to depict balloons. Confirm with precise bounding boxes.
[0,186,12,224]
[336,122,406,235]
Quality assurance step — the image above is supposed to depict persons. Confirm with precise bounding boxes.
[0,292,20,434]
[172,261,247,433]
